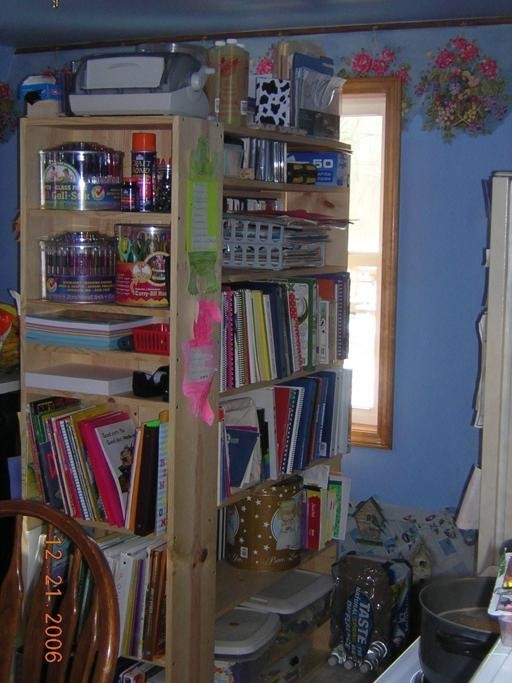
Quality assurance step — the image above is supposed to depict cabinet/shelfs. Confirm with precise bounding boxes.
[0,114,351,683]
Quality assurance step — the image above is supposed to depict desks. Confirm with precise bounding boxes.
[370,624,511,683]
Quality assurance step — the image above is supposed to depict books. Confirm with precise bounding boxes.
[220,273,351,393]
[271,36,347,141]
[223,138,351,271]
[26,396,168,682]
[217,368,350,561]
[24,309,154,396]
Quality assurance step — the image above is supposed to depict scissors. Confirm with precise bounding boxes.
[114,224,171,265]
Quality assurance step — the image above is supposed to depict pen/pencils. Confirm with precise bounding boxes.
[44,141,124,181]
[45,232,116,277]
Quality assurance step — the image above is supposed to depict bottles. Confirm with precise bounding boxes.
[120,133,156,214]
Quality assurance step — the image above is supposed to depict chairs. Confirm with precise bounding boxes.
[0,499,118,683]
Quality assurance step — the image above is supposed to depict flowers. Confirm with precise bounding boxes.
[331,37,511,145]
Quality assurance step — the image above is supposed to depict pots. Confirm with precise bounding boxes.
[418,577,501,683]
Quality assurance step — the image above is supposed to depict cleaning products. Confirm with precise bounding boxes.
[205,38,250,126]
